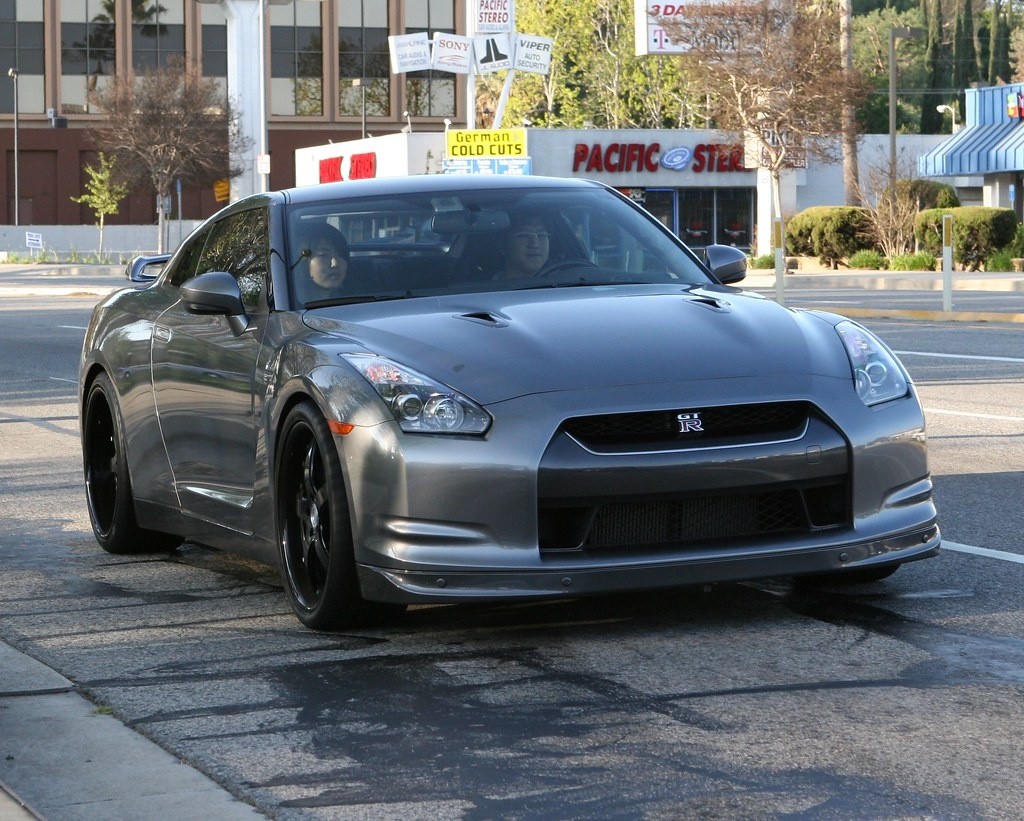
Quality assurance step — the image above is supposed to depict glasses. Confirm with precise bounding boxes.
[514,232,551,240]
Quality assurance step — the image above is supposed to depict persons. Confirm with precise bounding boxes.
[296,222,355,304]
[488,204,561,282]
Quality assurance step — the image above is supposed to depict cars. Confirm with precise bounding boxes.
[77,172,942,631]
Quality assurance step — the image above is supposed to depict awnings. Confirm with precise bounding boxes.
[917,82,1024,179]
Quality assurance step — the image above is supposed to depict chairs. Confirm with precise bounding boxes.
[450,208,576,283]
